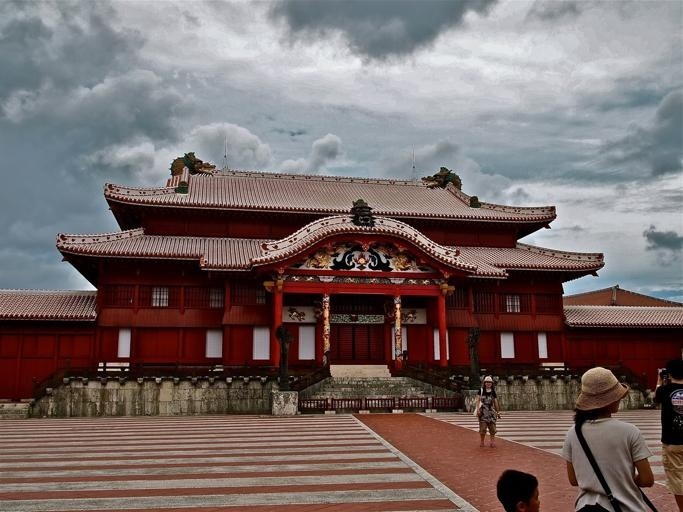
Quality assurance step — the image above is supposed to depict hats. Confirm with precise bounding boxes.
[483,376,494,384]
[575,368,629,412]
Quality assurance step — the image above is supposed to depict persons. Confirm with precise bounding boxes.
[652,357,683,511]
[561,366,654,511]
[475,375,502,449]
[496,469,541,512]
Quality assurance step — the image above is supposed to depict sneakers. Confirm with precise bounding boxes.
[480,442,495,448]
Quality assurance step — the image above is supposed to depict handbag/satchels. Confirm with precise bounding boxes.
[474,401,483,417]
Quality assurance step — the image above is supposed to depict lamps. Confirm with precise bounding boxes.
[181,262,186,278]
[130,285,134,303]
[349,298,358,322]
[206,270,212,279]
[136,268,141,277]
